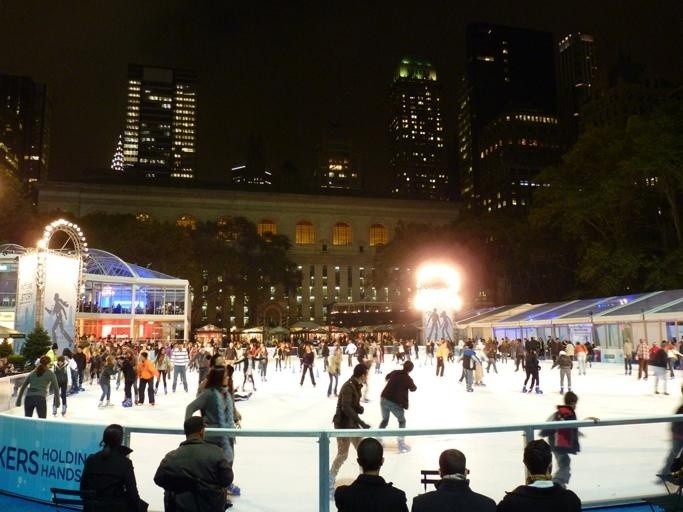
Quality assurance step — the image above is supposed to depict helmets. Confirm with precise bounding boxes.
[559,351,566,357]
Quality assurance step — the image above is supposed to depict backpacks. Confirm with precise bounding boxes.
[559,356,570,366]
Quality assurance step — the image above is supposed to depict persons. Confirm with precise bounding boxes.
[0,331,317,419]
[41,293,72,349]
[412,450,496,510]
[182,365,241,508]
[322,333,595,402]
[378,361,417,450]
[439,310,452,340]
[335,438,410,512]
[326,363,373,497]
[535,390,599,489]
[623,337,682,395]
[497,440,583,511]
[153,419,234,511]
[78,299,182,317]
[426,309,437,342]
[81,423,149,511]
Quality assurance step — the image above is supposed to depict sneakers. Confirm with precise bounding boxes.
[523,387,542,394]
[399,440,410,450]
[559,388,572,393]
[227,484,240,495]
[53,405,56,413]
[96,398,155,407]
[62,405,67,413]
[328,477,335,495]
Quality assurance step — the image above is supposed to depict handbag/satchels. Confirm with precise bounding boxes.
[539,412,561,436]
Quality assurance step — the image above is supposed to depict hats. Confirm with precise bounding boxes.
[184,416,208,433]
[52,343,58,349]
